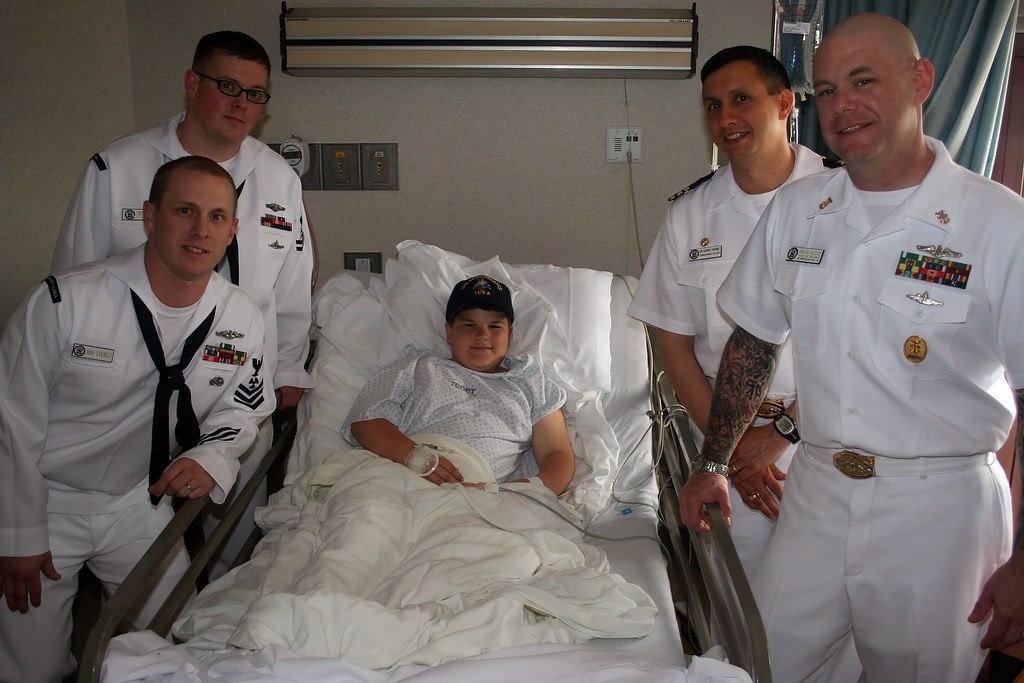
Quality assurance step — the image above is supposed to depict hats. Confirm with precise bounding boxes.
[446,275,514,326]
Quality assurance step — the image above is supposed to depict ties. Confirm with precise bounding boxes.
[130,288,216,506]
[212,180,245,286]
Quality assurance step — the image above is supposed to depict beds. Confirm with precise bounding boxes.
[79,273,774,683]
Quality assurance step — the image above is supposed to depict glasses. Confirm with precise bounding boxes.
[194,70,272,104]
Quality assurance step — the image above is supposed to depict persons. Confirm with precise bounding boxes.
[49,32,314,583]
[625,13,1024,683]
[0,155,277,683]
[341,274,576,495]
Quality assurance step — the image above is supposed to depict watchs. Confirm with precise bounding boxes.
[773,413,801,444]
[691,454,729,477]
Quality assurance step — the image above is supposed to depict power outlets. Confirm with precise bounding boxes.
[321,143,360,190]
[265,143,323,190]
[360,142,398,191]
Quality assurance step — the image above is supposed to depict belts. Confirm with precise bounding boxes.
[801,442,998,478]
[757,398,796,419]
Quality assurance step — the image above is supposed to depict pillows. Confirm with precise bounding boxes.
[396,238,613,395]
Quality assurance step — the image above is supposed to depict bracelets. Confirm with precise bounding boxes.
[523,476,544,489]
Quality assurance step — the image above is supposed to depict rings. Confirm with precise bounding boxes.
[732,465,738,471]
[185,482,193,491]
[749,493,759,501]
